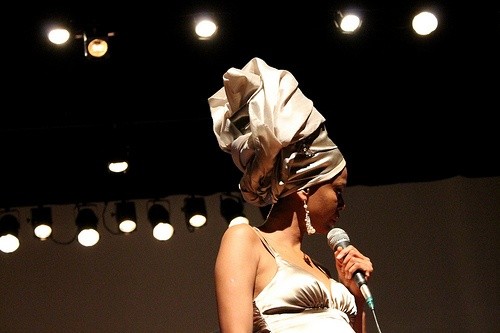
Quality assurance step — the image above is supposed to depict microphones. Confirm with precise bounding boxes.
[327,228,374,310]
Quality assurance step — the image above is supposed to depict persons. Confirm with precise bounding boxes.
[208,57,373,333]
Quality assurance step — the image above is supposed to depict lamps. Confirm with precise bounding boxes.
[181,195,208,233]
[146,198,175,242]
[0,208,21,254]
[27,206,53,241]
[219,191,250,228]
[110,200,137,233]
[81,25,113,62]
[71,202,100,247]
